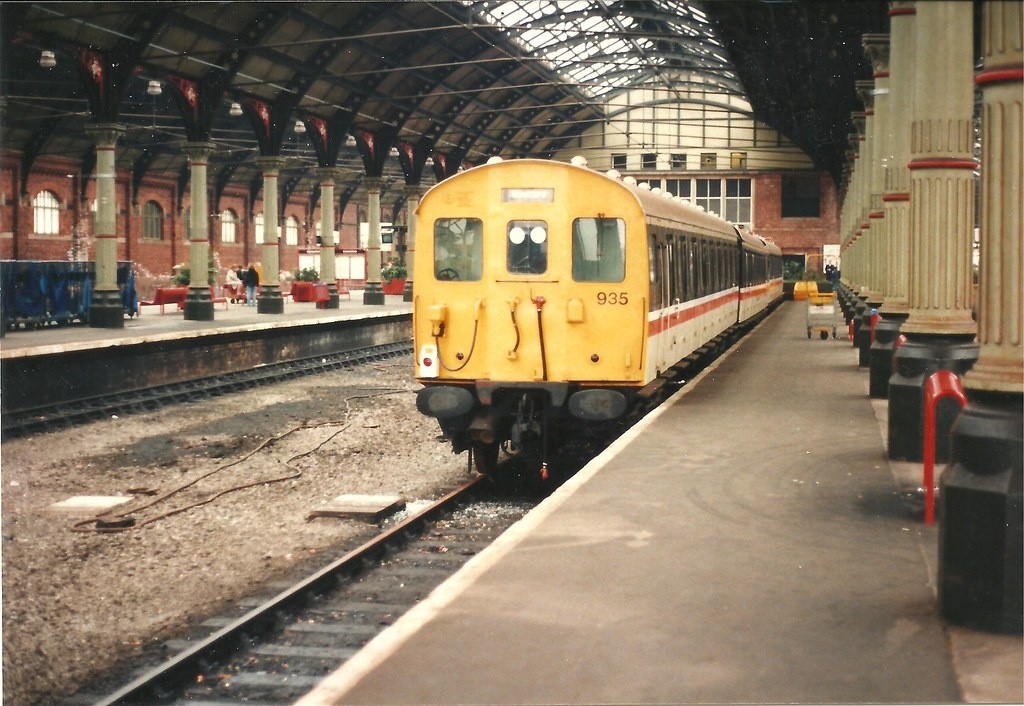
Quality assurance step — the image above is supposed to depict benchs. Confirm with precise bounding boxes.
[140,278,350,315]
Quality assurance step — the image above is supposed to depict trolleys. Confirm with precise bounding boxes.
[804,253,838,340]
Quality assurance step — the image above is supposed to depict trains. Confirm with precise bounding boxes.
[411,155,784,493]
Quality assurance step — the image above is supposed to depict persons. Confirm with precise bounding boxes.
[224,264,260,307]
[825,260,834,280]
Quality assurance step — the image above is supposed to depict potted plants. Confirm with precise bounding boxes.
[171,269,190,310]
[380,265,407,295]
[293,267,320,303]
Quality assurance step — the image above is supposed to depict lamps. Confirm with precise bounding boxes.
[425,157,434,166]
[294,121,306,132]
[389,146,400,156]
[229,103,243,115]
[40,50,56,67]
[147,80,162,95]
[458,165,464,172]
[346,135,356,146]
